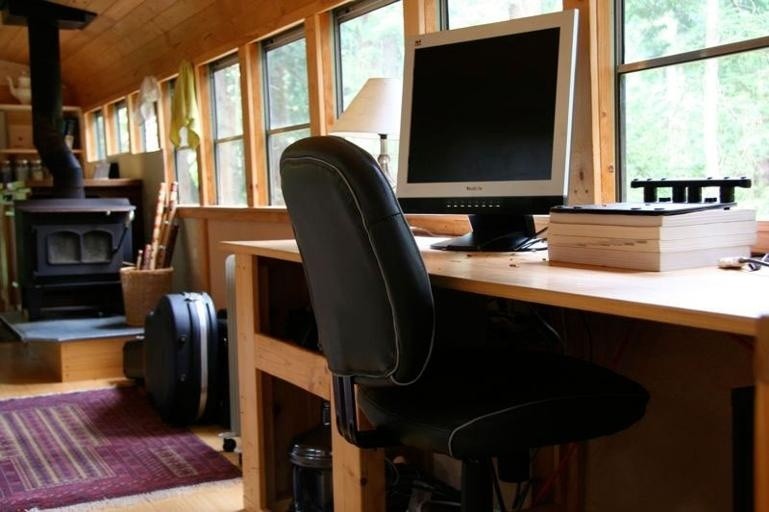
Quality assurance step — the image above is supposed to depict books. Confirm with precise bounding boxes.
[547,199,760,275]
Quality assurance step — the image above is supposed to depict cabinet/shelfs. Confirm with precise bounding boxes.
[0,104,88,312]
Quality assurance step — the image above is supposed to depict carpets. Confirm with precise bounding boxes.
[0,386,242,510]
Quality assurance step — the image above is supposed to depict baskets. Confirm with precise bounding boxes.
[119,263,175,328]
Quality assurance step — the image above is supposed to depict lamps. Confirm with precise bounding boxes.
[328,77,401,186]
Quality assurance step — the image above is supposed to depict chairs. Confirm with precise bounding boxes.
[280,135,648,511]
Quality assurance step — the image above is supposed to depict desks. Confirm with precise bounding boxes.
[220,231,769,510]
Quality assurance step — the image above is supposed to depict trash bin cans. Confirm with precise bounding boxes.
[289,430,333,512]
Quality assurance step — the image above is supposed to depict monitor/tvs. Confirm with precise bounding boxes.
[395,7,580,253]
